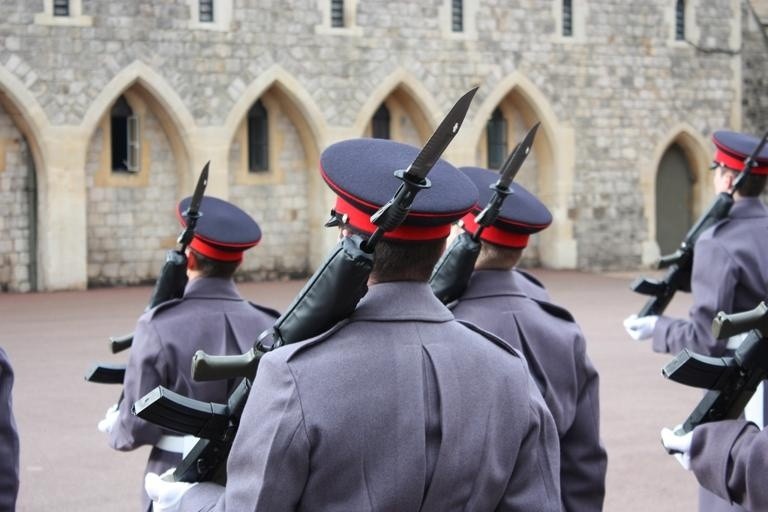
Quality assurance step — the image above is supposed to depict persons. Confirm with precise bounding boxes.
[99,194,284,512]
[144,138,564,512]
[624,131,768,427]
[441,164,608,512]
[659,419,768,512]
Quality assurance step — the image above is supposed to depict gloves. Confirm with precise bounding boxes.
[145,472,198,512]
[661,424,692,470]
[98,404,120,434]
[623,314,658,341]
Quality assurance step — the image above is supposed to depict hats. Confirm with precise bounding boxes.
[708,131,768,176]
[175,196,261,261]
[319,138,552,249]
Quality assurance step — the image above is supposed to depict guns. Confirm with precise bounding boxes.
[132,85,482,512]
[427,119,540,306]
[88,159,210,409]
[661,296,768,457]
[630,129,768,319]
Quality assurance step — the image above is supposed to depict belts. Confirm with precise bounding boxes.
[155,433,200,454]
[726,332,748,350]
